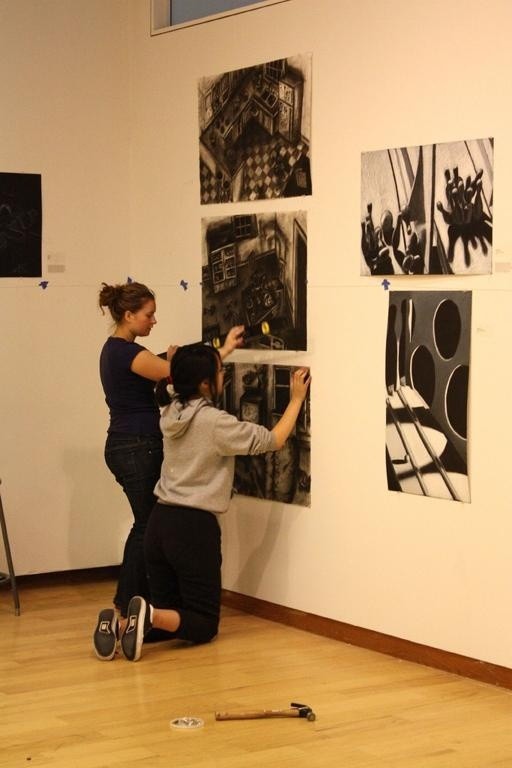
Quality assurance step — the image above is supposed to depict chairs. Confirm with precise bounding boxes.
[121,595,154,661]
[92,608,120,661]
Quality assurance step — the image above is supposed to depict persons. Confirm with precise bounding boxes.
[89,343,312,661]
[95,278,246,620]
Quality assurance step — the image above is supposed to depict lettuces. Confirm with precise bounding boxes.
[215,703,316,722]
